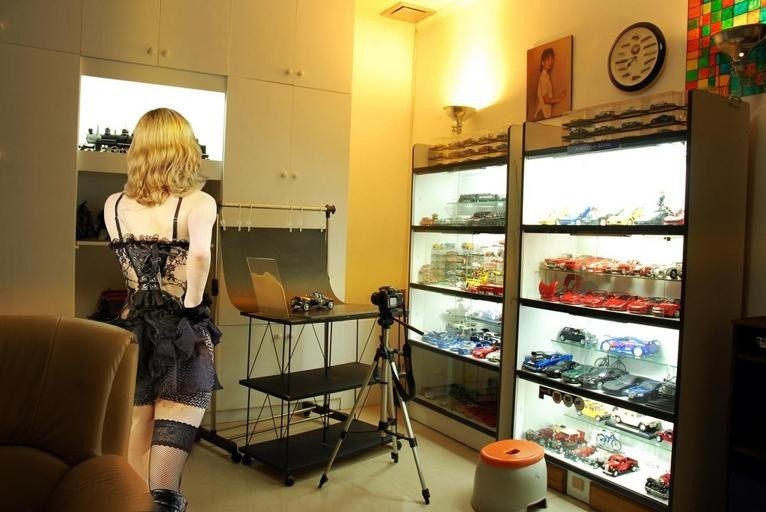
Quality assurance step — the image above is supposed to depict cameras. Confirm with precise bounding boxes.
[371,286,404,309]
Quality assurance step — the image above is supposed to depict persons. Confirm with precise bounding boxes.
[532,47,567,120]
[291,291,336,311]
[99,106,226,512]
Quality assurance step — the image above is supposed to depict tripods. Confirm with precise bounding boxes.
[319,310,430,505]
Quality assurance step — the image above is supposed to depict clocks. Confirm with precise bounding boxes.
[607,22,667,94]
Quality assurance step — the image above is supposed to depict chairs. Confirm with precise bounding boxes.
[1,312,162,512]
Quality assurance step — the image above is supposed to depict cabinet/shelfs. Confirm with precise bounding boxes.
[239,302,401,488]
